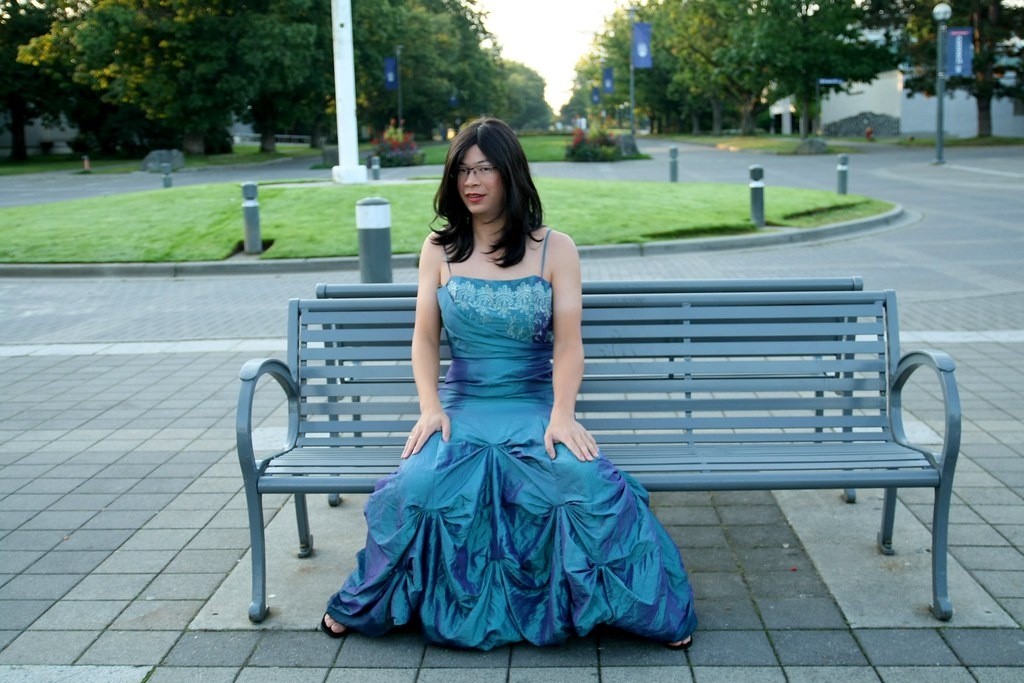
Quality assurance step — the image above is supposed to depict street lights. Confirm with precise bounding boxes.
[932,2,952,165]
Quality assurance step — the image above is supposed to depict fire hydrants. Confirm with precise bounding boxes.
[865,127,873,139]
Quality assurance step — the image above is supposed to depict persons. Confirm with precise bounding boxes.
[321,119,698,650]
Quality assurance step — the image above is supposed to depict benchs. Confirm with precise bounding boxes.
[235,274,962,624]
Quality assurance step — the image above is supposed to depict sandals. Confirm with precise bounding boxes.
[661,634,693,651]
[321,611,353,638]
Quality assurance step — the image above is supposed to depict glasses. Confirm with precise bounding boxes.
[457,165,497,178]
